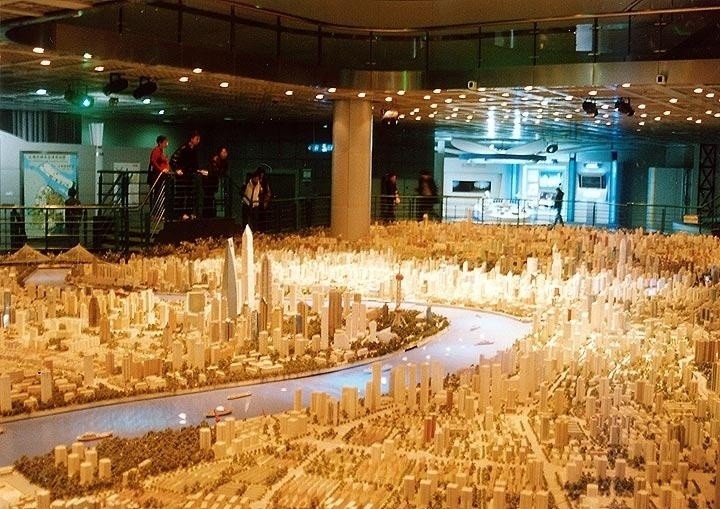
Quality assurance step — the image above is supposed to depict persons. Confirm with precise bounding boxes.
[203,146,231,217]
[146,133,172,220]
[380,170,400,223]
[169,128,208,220]
[551,186,565,226]
[414,167,442,223]
[251,167,272,228]
[63,187,83,245]
[240,168,264,230]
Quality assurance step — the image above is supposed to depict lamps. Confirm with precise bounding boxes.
[613,96,636,117]
[63,70,156,108]
[581,95,599,117]
